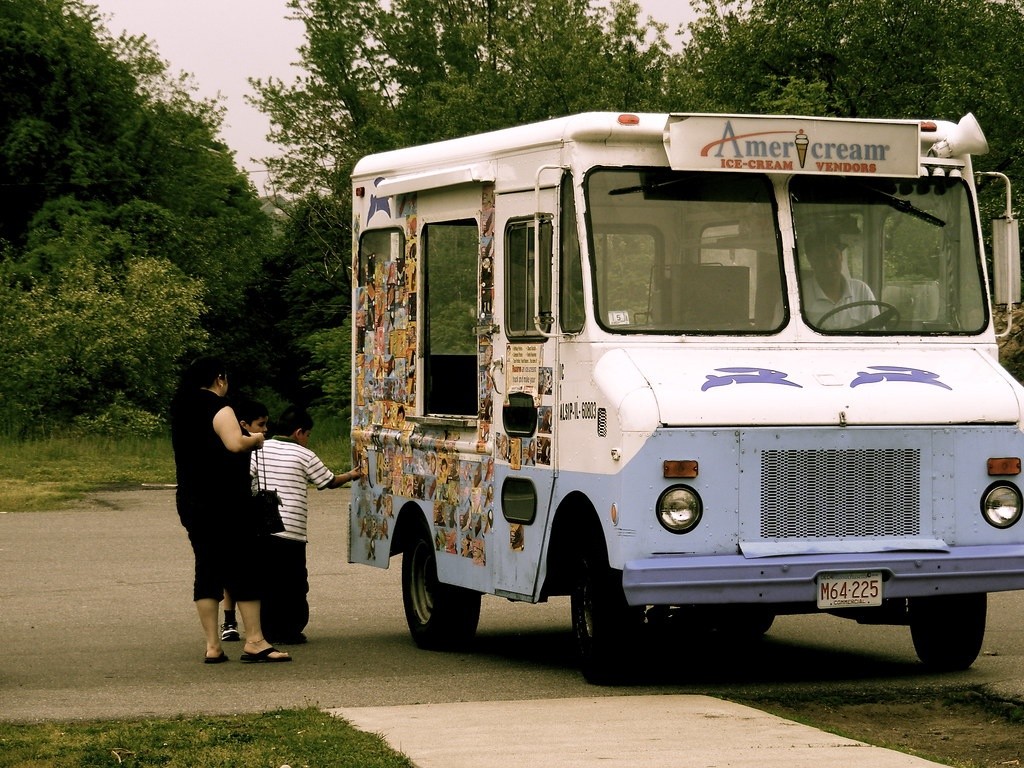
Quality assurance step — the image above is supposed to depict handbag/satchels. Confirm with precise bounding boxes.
[251,448,287,534]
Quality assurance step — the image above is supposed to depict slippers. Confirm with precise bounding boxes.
[205,650,228,663]
[240,648,292,662]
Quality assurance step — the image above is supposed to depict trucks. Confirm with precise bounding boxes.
[344,109,1023,687]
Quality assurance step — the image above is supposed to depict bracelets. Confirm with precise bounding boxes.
[346,472,352,482]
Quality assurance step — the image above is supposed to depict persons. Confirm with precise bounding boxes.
[221,400,364,644]
[772,227,885,331]
[167,357,292,662]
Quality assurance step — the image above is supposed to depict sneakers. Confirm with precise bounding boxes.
[220,622,240,642]
